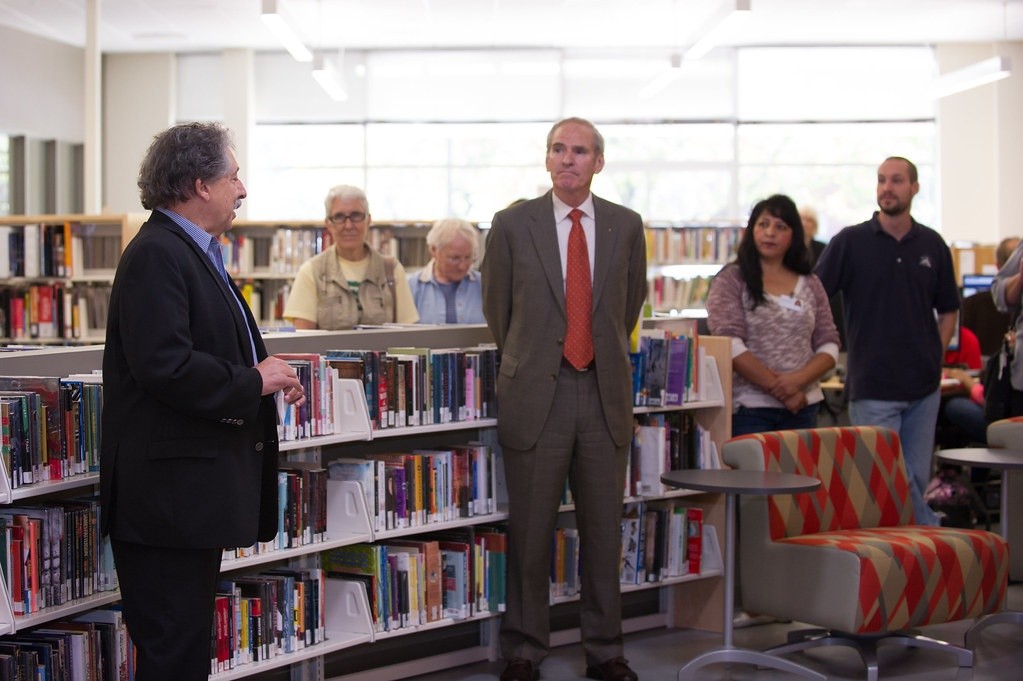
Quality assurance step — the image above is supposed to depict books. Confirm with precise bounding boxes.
[549,330,726,602]
[219,229,397,328]
[645,227,743,318]
[0,610,136,681]
[210,342,506,676]
[0,500,119,616]
[0,370,101,489]
[0,224,119,351]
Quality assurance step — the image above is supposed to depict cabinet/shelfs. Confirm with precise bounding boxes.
[0,212,740,681]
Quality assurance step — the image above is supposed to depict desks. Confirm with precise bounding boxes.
[818,375,981,396]
[658,463,828,681]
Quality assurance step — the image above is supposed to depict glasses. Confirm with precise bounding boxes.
[326,209,367,224]
[444,252,475,263]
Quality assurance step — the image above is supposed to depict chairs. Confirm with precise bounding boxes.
[982,415,1023,592]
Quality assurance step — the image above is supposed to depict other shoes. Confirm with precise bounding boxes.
[498,658,543,680]
[584,653,639,681]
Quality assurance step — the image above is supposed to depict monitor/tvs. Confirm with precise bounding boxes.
[963,274,994,299]
[934,304,962,352]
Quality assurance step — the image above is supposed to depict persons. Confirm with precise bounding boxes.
[99,122,307,681]
[706,195,847,437]
[480,119,647,681]
[936,237,1023,525]
[283,184,419,330]
[811,156,964,527]
[407,218,487,324]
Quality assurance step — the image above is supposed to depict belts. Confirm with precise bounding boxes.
[555,350,595,371]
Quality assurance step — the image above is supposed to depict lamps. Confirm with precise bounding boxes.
[684,0,751,62]
[931,0,1014,101]
[261,0,351,102]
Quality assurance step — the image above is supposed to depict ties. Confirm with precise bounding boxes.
[559,208,593,371]
[209,236,229,285]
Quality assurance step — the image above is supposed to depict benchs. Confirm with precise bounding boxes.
[928,446,1023,661]
[724,425,1009,681]
